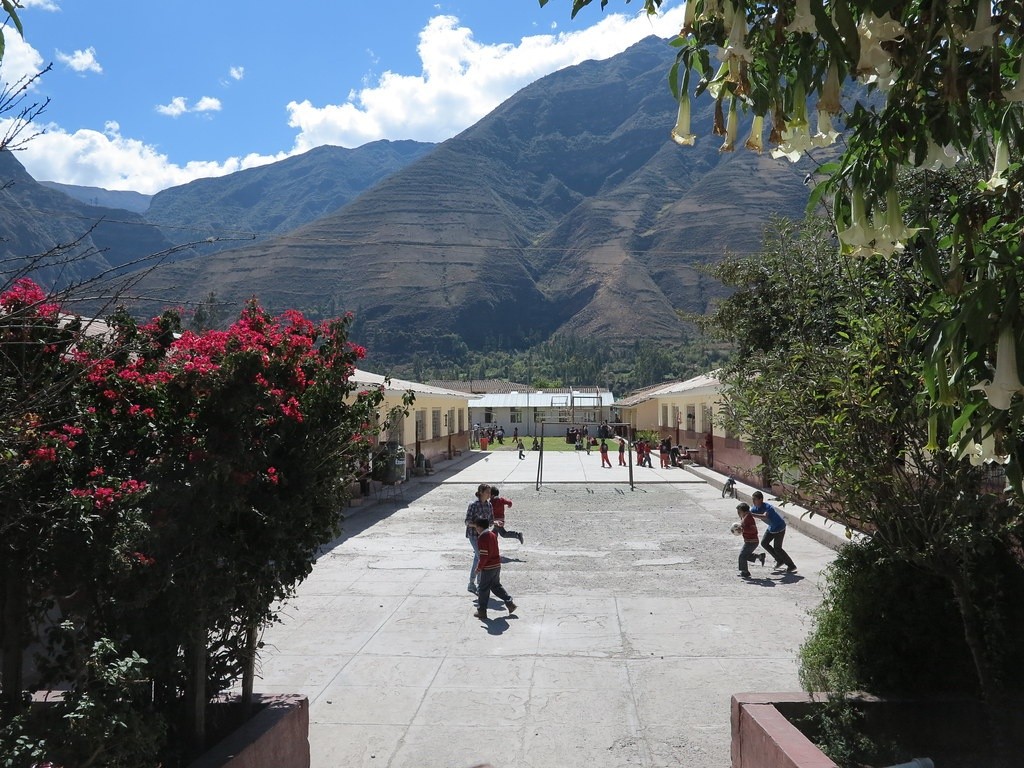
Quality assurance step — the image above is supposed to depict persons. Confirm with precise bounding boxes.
[591,438,599,446]
[586,442,591,454]
[634,438,652,468]
[465,484,504,595]
[473,422,505,446]
[517,439,525,459]
[735,503,766,577]
[473,518,517,619]
[700,434,713,467]
[567,424,589,450]
[533,437,540,450]
[618,439,626,466]
[512,427,519,443]
[750,491,797,573]
[656,435,690,469]
[489,486,523,544]
[600,439,612,467]
[598,419,615,439]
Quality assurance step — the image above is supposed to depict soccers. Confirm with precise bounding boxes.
[730,523,743,536]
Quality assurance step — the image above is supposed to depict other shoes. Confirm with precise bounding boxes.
[775,562,783,568]
[760,553,765,566]
[737,572,750,577]
[509,604,517,613]
[518,533,523,544]
[786,565,797,572]
[474,611,487,618]
[468,584,477,592]
